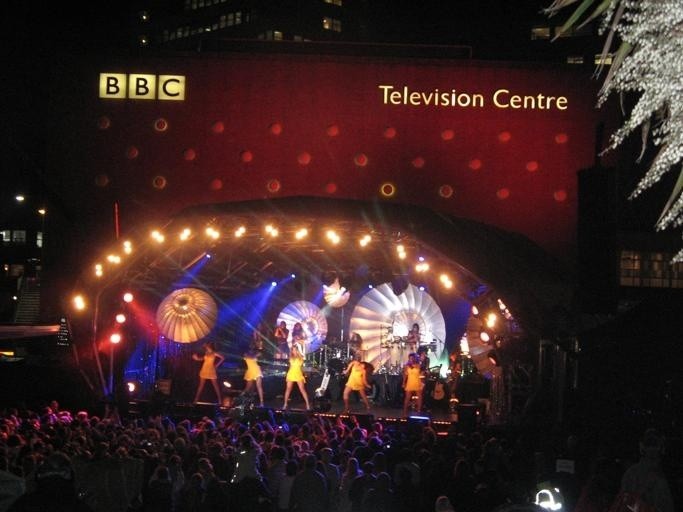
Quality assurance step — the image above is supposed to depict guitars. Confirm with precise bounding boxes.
[434,365,444,400]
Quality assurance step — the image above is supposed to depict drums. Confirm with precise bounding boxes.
[327,348,346,369]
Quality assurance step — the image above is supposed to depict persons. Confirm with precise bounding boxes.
[275,321,289,359]
[280,342,309,411]
[190,341,225,404]
[235,342,264,407]
[339,354,371,414]
[292,322,308,353]
[401,353,425,419]
[379,335,402,372]
[348,332,363,361]
[1,400,682,512]
[406,323,419,360]
[254,321,270,349]
[446,353,461,408]
[415,345,430,374]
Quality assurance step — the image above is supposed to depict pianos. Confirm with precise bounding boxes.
[381,341,437,352]
